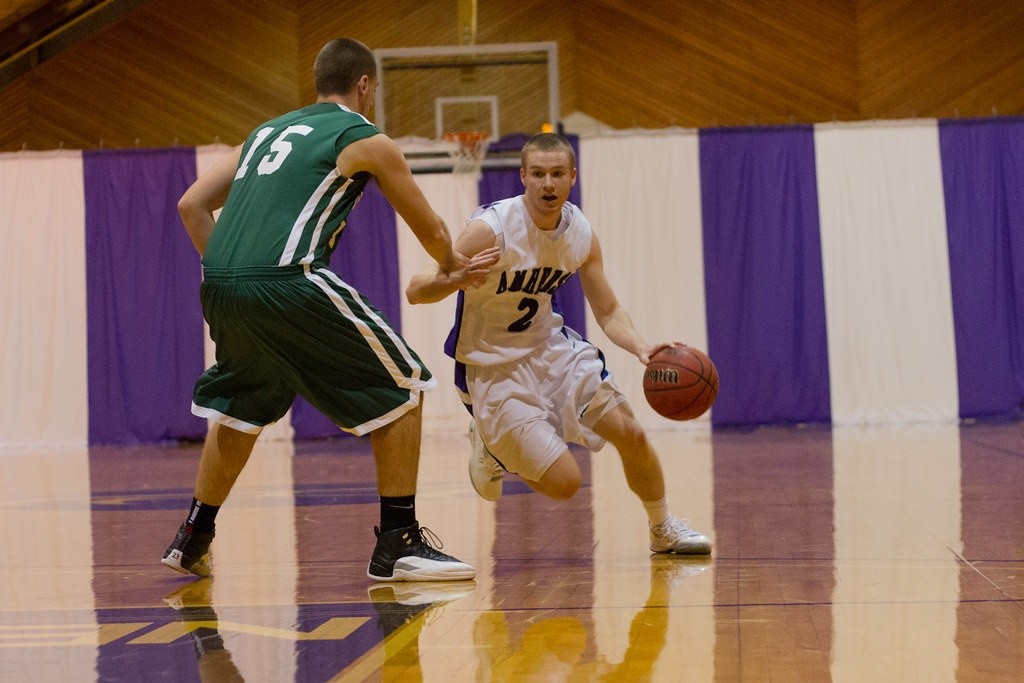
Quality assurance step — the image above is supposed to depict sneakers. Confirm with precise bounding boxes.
[649,516,711,556]
[367,522,477,581]
[161,520,215,577]
[468,416,504,501]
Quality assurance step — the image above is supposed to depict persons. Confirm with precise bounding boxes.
[156,32,501,581]
[407,130,715,556]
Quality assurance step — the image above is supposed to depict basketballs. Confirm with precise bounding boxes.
[641,342,721,422]
